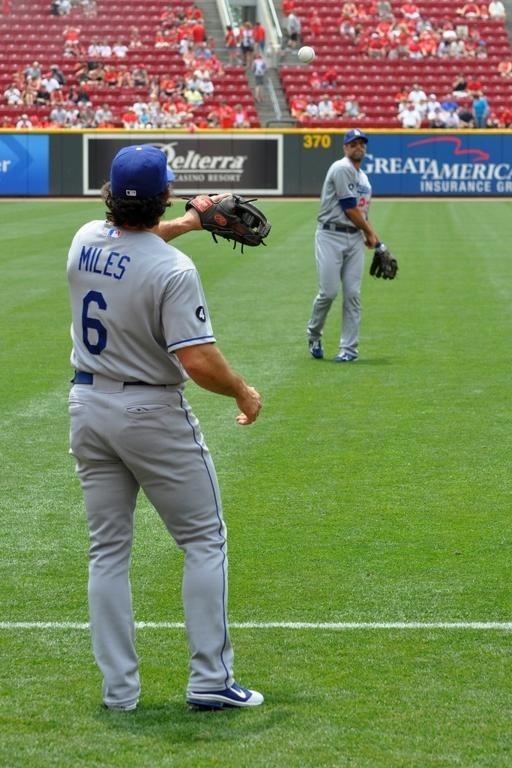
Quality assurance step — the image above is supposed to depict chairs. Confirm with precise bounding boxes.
[1,1,512,128]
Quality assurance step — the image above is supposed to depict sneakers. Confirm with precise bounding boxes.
[186,682,264,708]
[308,340,323,359]
[334,352,354,362]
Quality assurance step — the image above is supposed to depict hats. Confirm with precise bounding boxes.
[110,144,176,199]
[343,128,368,144]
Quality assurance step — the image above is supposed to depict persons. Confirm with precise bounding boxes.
[64,144,265,714]
[305,128,381,364]
[226,21,265,104]
[4,24,130,129]
[287,1,512,127]
[55,0,99,20]
[121,5,250,128]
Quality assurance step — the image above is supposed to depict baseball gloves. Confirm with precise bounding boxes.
[370,242,397,280]
[184,193,271,246]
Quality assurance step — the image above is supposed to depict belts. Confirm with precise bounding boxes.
[73,371,164,387]
[323,223,358,233]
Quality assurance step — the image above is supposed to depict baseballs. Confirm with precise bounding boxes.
[297,45,316,63]
